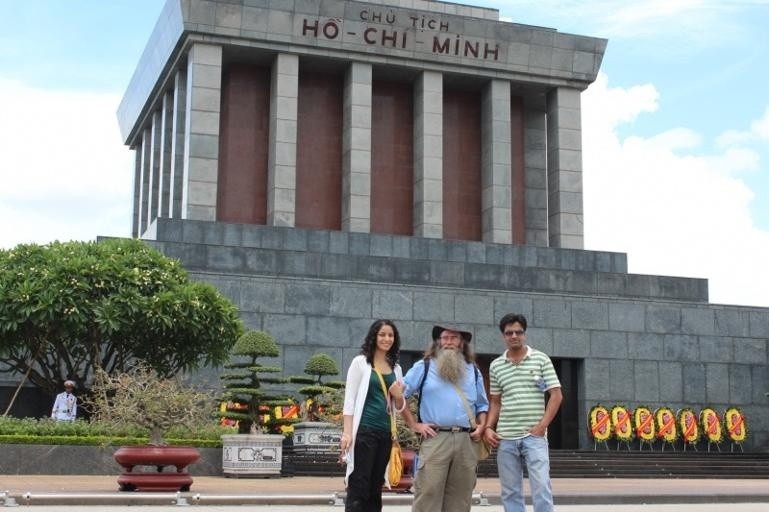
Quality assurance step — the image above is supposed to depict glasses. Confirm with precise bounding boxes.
[503,328,525,336]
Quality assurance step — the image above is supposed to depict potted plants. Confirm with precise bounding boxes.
[78,360,212,492]
[217,329,347,477]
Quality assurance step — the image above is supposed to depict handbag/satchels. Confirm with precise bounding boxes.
[388,439,403,487]
[479,437,493,461]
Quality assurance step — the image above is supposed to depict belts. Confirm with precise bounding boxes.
[432,426,476,433]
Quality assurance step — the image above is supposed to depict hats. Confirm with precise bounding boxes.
[432,322,472,344]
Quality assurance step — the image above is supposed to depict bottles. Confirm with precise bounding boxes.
[534,375,546,390]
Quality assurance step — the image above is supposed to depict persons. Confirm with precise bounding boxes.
[401,322,489,512]
[340,319,404,512]
[51,380,77,421]
[482,312,564,512]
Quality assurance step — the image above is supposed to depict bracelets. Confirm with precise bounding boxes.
[484,424,494,430]
